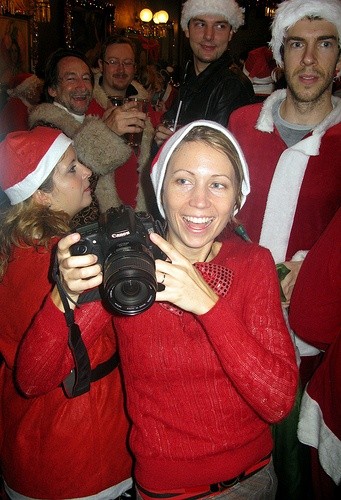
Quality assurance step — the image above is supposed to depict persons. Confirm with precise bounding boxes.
[0,124,135,499]
[14,120,300,500]
[1,72,50,142]
[152,0,256,151]
[224,0,341,499]
[27,48,147,232]
[287,195,341,500]
[84,35,166,207]
[0,28,341,103]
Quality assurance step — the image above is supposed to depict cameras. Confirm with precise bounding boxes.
[70,205,165,315]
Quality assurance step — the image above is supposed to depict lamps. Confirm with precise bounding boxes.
[139,8,171,39]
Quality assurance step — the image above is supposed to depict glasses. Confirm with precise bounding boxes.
[102,58,136,69]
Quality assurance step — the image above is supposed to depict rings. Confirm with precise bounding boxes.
[160,273,166,284]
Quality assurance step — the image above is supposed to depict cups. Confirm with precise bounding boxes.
[110,96,122,107]
[121,99,148,146]
[163,120,186,131]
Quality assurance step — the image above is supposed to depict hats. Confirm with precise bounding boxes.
[181,0,244,34]
[7,71,40,97]
[0,126,73,205]
[268,0,341,69]
[150,118,251,221]
[243,47,275,84]
